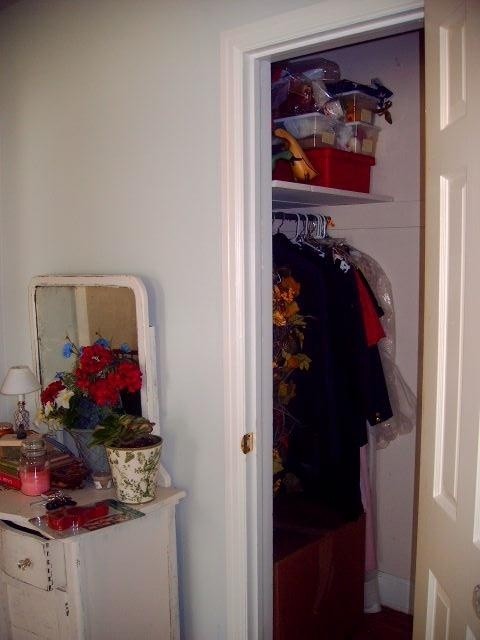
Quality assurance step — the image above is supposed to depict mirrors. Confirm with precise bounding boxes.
[28,275,155,432]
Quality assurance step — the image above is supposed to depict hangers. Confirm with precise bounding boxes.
[272,210,335,246]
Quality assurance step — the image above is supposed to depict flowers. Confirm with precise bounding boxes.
[33,332,144,430]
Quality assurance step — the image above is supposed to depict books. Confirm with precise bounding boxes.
[0,433,33,446]
[0,457,77,478]
[0,453,70,470]
[0,472,26,491]
[0,440,46,458]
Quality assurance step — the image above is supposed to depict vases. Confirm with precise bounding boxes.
[68,426,113,476]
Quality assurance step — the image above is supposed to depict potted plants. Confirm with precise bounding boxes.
[87,407,162,505]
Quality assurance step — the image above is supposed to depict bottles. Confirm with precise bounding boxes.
[17,435,50,498]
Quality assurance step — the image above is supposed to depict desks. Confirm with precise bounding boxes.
[0,483,188,640]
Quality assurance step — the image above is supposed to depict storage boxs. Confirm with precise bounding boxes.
[272,59,383,194]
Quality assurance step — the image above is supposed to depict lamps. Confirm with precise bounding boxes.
[0,364,42,447]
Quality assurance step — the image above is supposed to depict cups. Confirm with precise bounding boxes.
[91,470,113,490]
